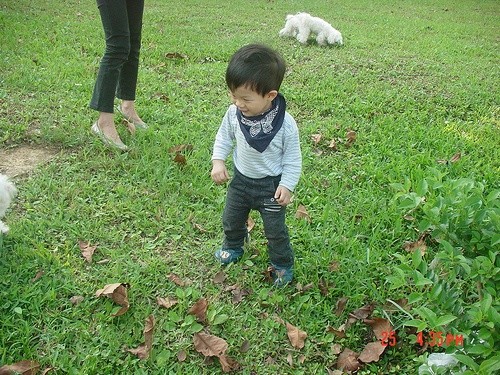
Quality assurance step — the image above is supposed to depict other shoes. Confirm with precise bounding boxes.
[214,247,244,264]
[270,260,294,285]
[91,120,129,152]
[116,106,150,129]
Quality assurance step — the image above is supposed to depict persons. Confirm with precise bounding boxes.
[89,0,149,152]
[210,44,302,285]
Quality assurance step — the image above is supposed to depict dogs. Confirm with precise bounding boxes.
[0,173,19,233]
[279,11,343,47]
